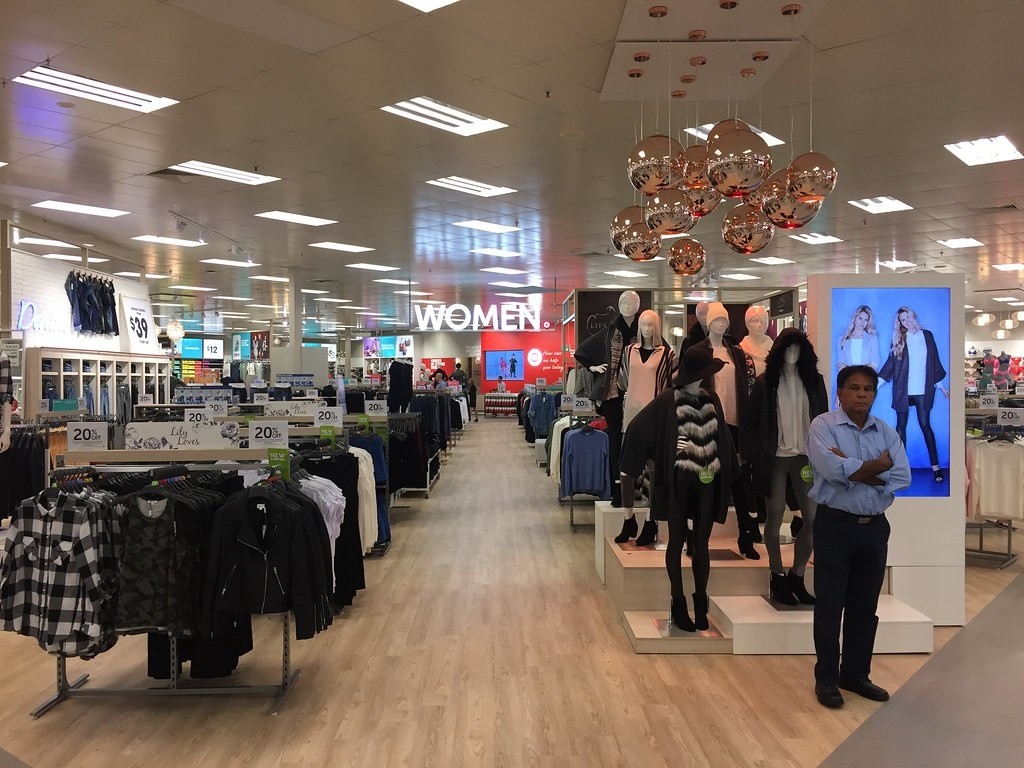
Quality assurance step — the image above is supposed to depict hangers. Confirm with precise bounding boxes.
[965,386,1024,445]
[0,373,470,515]
[518,385,603,434]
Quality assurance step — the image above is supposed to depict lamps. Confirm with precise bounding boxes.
[171,295,223,319]
[607,0,842,274]
[196,230,204,244]
[970,299,1024,340]
[176,219,188,232]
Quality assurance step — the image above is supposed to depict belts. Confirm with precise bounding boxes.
[817,504,884,525]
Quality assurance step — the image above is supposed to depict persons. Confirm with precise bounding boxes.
[456,372,471,423]
[420,363,467,386]
[738,327,828,610]
[879,306,949,482]
[0,336,13,452]
[973,349,1016,390]
[492,376,509,417]
[499,353,517,377]
[834,305,880,409]
[808,364,912,708]
[620,345,733,632]
[572,290,804,560]
[468,378,478,422]
[435,373,446,390]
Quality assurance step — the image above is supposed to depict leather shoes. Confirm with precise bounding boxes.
[815,682,844,706]
[837,677,889,702]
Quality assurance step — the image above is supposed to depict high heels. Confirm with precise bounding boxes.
[692,593,709,631]
[769,572,798,606]
[786,569,816,605]
[671,596,696,632]
[750,515,762,543]
[635,518,658,546]
[738,536,760,560]
[790,516,804,538]
[611,483,622,507]
[614,514,638,543]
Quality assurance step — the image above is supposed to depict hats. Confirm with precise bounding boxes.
[671,344,724,385]
[706,302,729,331]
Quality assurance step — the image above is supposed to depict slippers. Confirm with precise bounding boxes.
[933,467,943,483]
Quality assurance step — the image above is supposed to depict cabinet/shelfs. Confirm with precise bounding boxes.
[26,347,171,420]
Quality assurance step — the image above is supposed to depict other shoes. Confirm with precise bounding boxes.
[504,414,508,416]
[474,419,478,422]
